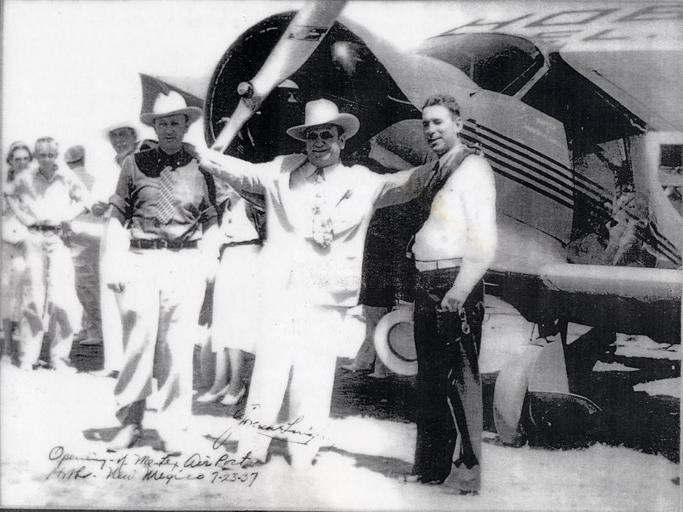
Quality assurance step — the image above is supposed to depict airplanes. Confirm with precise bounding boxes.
[64,0,681,437]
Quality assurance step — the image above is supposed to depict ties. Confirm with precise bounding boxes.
[157,158,177,224]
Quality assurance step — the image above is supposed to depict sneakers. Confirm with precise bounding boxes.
[105,423,143,452]
[79,337,101,346]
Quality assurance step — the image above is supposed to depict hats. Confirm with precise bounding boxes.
[139,90,204,126]
[285,98,362,142]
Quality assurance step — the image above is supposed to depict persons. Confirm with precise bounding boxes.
[406,94,499,495]
[103,88,223,460]
[131,98,488,479]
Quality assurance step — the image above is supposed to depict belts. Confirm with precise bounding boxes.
[130,238,199,250]
[416,258,463,273]
[31,225,62,231]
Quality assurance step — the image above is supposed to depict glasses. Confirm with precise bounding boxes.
[306,131,334,140]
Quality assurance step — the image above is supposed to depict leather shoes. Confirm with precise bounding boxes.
[220,383,247,406]
[197,382,230,403]
